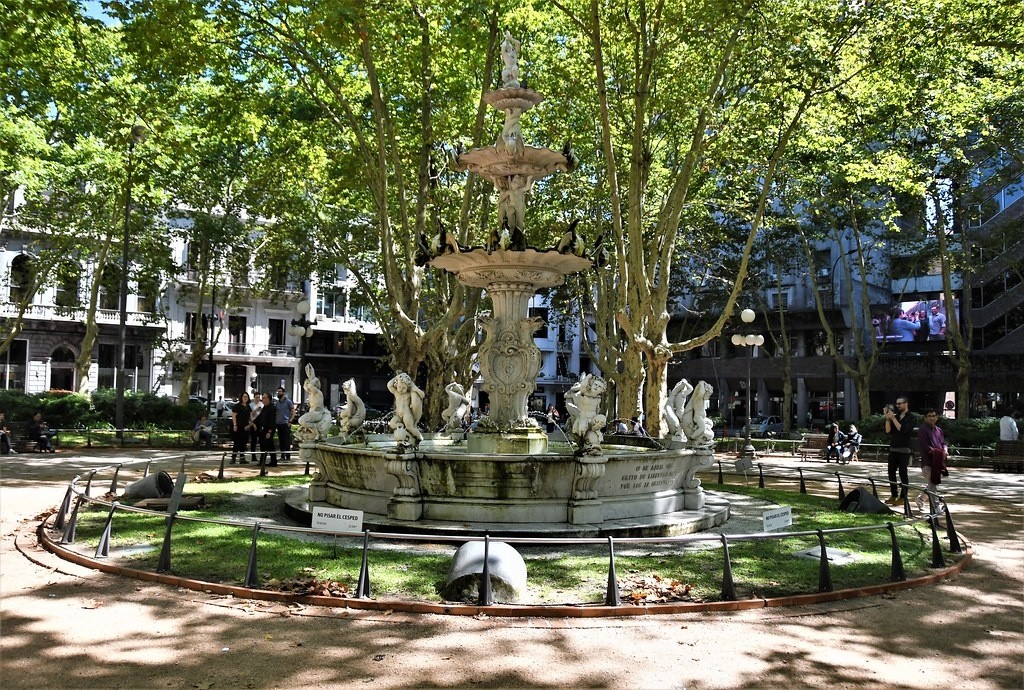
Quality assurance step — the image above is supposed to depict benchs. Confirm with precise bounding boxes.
[0,422,48,454]
[191,418,260,451]
[797,433,863,462]
[990,440,1024,474]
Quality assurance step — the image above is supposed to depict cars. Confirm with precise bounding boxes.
[364,409,390,432]
[293,403,311,423]
[741,416,784,438]
[188,398,236,418]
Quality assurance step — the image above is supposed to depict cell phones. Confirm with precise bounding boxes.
[911,312,915,315]
[42,422,47,425]
[887,404,894,410]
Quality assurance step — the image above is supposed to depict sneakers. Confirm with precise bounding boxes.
[9,449,18,455]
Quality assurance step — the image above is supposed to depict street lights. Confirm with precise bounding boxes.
[731,309,764,459]
[115,126,150,439]
[288,301,319,416]
[830,249,858,422]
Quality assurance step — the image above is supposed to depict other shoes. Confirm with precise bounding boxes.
[916,499,926,511]
[230,456,235,464]
[280,458,285,462]
[284,459,290,463]
[240,458,249,464]
[897,498,904,507]
[265,462,277,467]
[885,497,896,506]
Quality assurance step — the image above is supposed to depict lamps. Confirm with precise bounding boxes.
[251,372,258,382]
[219,371,225,381]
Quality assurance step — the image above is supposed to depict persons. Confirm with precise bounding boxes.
[216,396,226,420]
[889,304,947,341]
[496,30,533,238]
[915,407,949,516]
[674,378,713,445]
[757,409,764,416]
[806,409,816,431]
[298,378,470,441]
[29,411,57,453]
[826,423,863,464]
[543,404,560,433]
[0,411,18,454]
[194,386,295,467]
[1000,407,1019,441]
[883,397,917,506]
[613,417,647,436]
[563,375,608,448]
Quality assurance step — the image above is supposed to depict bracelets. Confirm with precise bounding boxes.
[288,421,292,423]
[268,432,271,434]
[233,425,237,426]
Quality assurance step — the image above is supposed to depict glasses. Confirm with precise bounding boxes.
[896,402,907,405]
[928,415,936,417]
